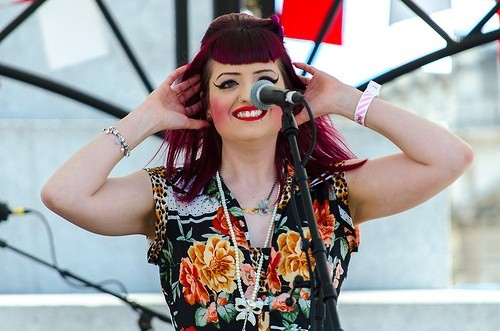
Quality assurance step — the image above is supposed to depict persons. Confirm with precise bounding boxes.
[40,13,473,331]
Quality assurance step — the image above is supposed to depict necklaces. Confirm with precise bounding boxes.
[215,170,281,326]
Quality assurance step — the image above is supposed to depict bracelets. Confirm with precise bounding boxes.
[101,126,132,158]
[354,80,382,127]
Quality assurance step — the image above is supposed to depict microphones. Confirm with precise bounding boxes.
[250,80,306,110]
